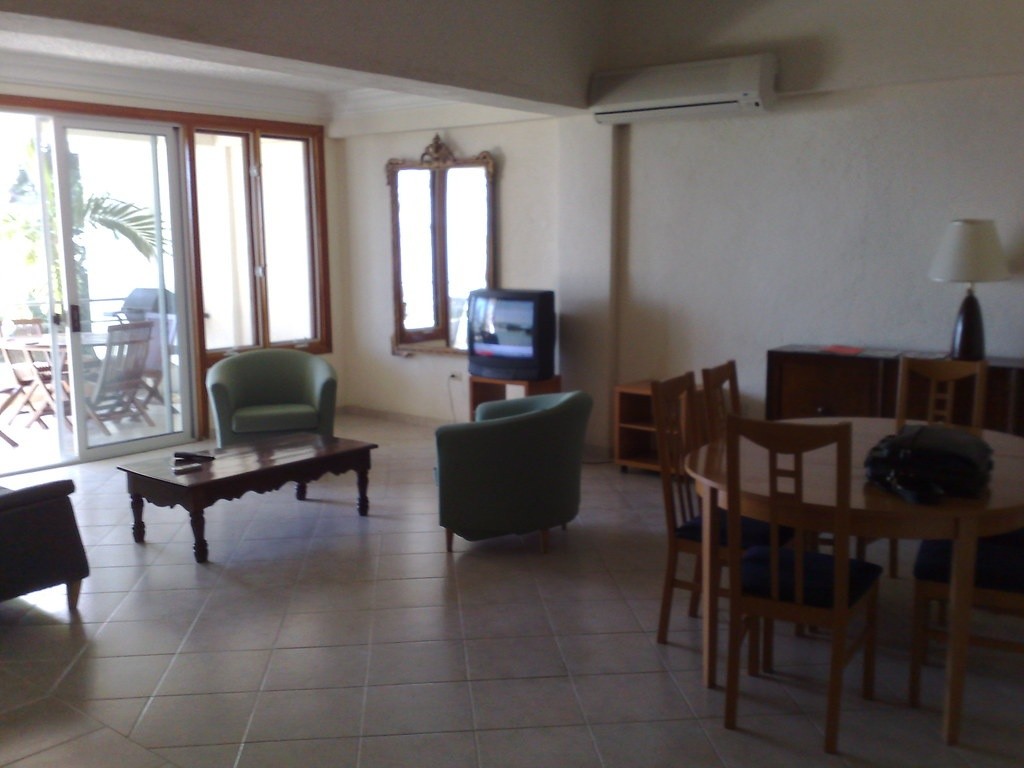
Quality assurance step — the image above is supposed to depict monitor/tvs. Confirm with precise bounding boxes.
[467,289,555,380]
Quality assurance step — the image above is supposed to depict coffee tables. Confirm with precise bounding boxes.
[116,435,379,563]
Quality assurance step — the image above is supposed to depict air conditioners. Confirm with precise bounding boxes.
[587,54,781,128]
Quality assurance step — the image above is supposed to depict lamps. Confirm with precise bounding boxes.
[922,218,1010,358]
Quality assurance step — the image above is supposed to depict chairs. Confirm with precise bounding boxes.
[684,360,818,616]
[645,368,789,644]
[1,311,181,450]
[896,357,987,426]
[435,388,595,554]
[0,476,90,614]
[906,528,1023,712]
[706,418,884,753]
[207,347,339,451]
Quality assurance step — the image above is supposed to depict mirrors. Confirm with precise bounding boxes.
[383,134,498,355]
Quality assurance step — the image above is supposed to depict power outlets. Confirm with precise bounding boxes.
[451,370,461,381]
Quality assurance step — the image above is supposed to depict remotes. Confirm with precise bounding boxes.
[171,464,201,474]
[174,452,214,462]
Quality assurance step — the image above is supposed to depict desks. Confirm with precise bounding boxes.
[684,416,1024,746]
[1,334,150,438]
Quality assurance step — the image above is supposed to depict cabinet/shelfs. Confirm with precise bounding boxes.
[615,381,711,473]
[762,344,1024,436]
[467,374,563,428]
